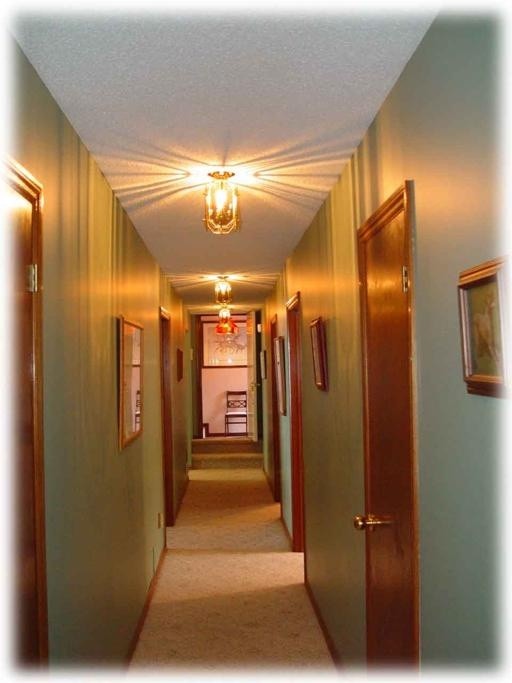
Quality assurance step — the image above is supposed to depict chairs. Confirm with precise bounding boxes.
[224,390,248,438]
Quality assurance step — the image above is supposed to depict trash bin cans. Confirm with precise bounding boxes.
[200,422,209,437]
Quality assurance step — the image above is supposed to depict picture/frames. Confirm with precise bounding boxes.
[273,336,287,417]
[309,316,329,392]
[117,312,145,453]
[201,320,248,368]
[456,255,510,400]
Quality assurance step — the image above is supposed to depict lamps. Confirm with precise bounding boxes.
[201,171,242,236]
[213,275,233,306]
